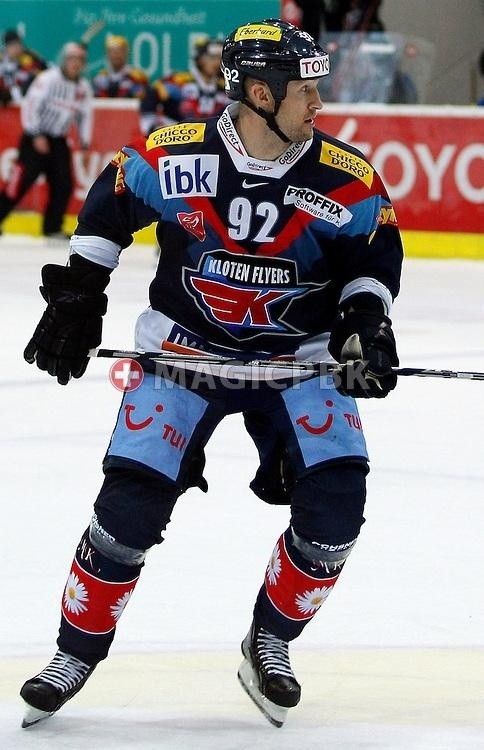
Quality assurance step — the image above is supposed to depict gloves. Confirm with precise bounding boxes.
[327,292,399,398]
[24,263,110,384]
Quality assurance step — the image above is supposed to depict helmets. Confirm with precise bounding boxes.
[191,33,225,62]
[220,19,331,102]
[107,35,127,50]
[63,41,86,63]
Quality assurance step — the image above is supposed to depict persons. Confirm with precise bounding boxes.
[19,18,403,712]
[0,0,418,241]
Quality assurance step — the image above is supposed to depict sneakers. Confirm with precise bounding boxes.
[20,649,96,712]
[240,617,300,708]
